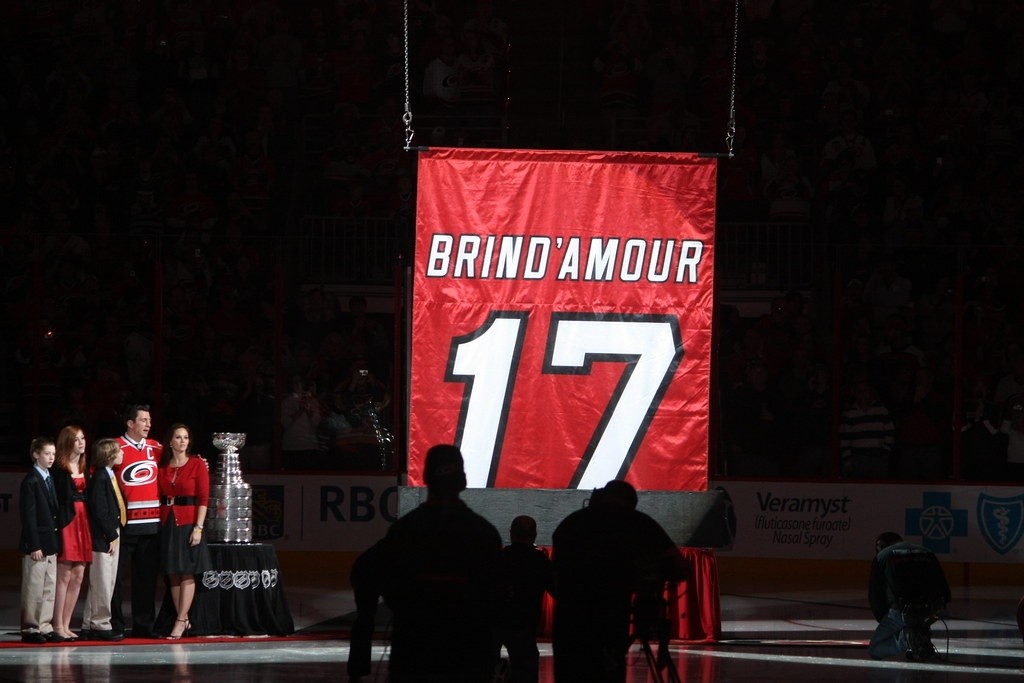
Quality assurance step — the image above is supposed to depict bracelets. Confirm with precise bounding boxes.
[197,524,203,529]
[194,528,202,533]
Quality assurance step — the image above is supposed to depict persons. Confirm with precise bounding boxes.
[496,516,554,683]
[866,531,950,661]
[717,141,1024,482]
[413,0,1023,152]
[552,480,693,683]
[49,425,93,642]
[347,445,504,683]
[85,438,128,640]
[20,436,64,643]
[1,0,413,471]
[157,426,210,640]
[90,403,209,639]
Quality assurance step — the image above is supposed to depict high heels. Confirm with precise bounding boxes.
[166,619,192,640]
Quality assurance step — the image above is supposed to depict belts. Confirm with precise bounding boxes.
[159,494,199,507]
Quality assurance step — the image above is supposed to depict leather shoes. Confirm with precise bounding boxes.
[56,633,80,641]
[81,629,124,640]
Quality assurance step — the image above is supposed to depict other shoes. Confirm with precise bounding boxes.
[42,632,64,641]
[132,630,161,638]
[21,633,46,644]
[899,628,935,661]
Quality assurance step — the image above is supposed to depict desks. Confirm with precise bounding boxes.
[536,545,722,642]
[156,544,296,637]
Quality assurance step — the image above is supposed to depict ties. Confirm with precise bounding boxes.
[111,472,127,527]
[46,477,57,521]
